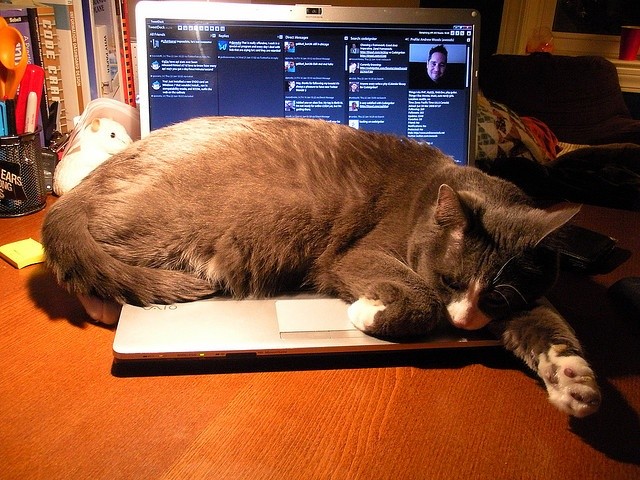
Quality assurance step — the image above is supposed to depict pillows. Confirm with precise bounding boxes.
[520,116,562,160]
[475,96,544,171]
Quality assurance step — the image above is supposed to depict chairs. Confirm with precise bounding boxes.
[491,57,640,144]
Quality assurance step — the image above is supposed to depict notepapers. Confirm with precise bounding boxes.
[0,238,47,269]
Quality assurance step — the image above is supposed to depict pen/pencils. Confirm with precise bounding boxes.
[0,103,8,138]
[22,91,37,135]
[5,99,17,137]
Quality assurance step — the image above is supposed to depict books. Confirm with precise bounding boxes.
[0,0,139,135]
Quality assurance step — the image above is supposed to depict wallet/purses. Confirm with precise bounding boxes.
[561,225,618,274]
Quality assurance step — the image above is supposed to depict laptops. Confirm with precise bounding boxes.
[113,1,503,360]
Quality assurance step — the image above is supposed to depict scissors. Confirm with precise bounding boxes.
[1,15,25,98]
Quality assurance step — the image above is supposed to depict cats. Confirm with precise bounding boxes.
[40,113,604,420]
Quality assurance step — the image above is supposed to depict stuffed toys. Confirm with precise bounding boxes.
[53,118,133,198]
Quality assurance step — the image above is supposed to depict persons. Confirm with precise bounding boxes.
[410,44,465,89]
[349,44,360,53]
[286,81,296,92]
[349,83,359,93]
[288,42,295,53]
[287,61,296,72]
[349,62,360,74]
[349,102,359,111]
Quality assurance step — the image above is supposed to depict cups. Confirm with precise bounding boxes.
[619,25,640,62]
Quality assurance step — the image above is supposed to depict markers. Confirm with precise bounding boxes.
[15,63,47,137]
